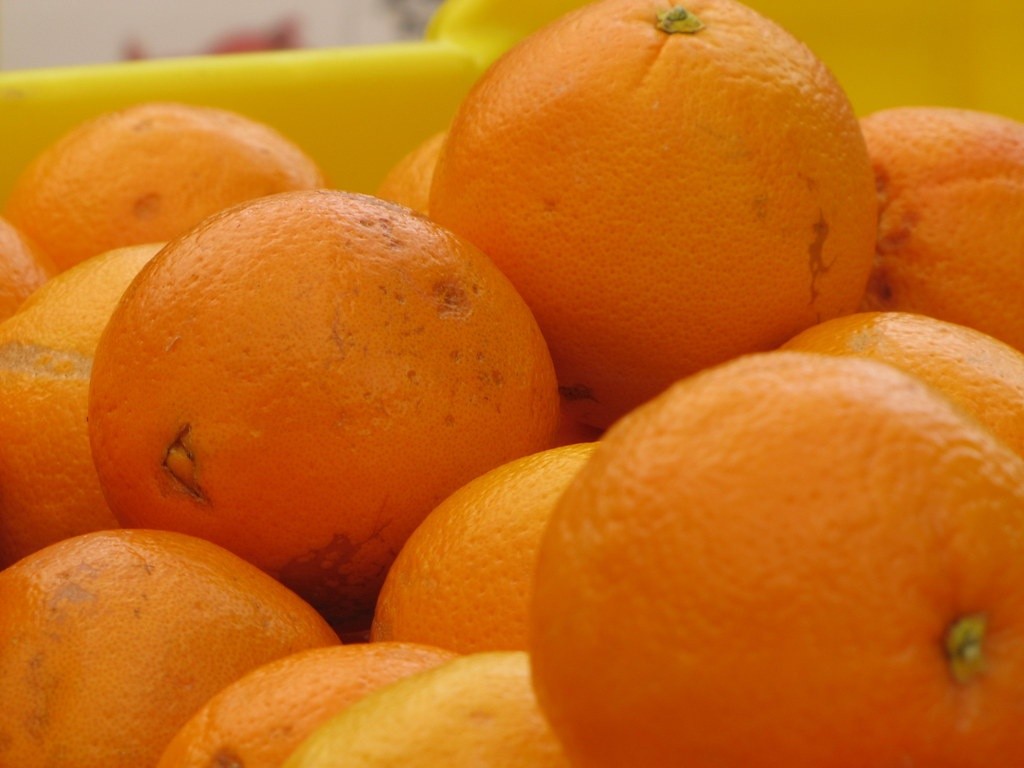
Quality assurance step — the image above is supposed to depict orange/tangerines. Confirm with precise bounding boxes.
[1,0,1024,768]
